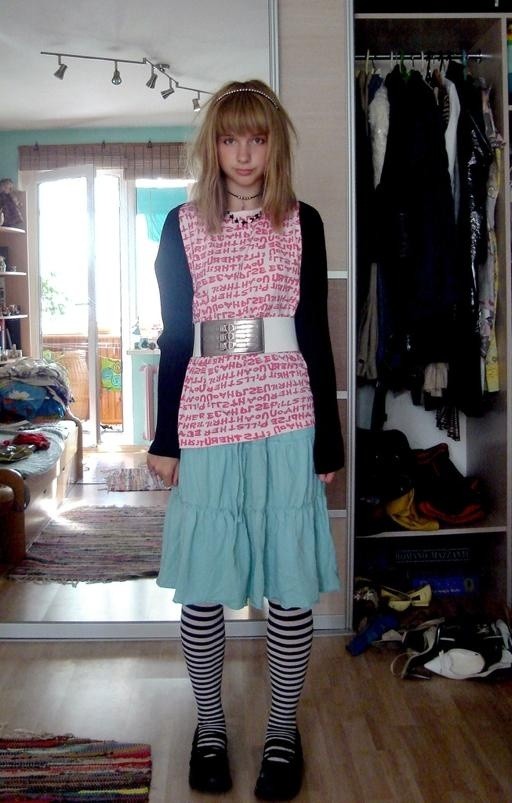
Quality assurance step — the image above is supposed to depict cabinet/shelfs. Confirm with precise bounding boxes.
[0,181,34,361]
[337,0,509,639]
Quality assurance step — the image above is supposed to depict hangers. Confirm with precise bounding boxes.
[360,45,479,85]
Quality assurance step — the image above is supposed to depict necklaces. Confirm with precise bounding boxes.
[227,188,264,212]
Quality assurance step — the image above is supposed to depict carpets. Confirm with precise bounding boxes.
[9,502,168,585]
[0,722,152,802]
[99,458,175,491]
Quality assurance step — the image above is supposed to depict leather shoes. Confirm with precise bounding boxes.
[253,726,306,803]
[186,722,235,796]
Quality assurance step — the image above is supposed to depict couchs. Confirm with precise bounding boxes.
[0,354,83,567]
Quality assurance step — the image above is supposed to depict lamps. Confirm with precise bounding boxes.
[43,47,213,112]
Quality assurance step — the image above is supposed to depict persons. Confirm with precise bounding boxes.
[145,78,347,802]
